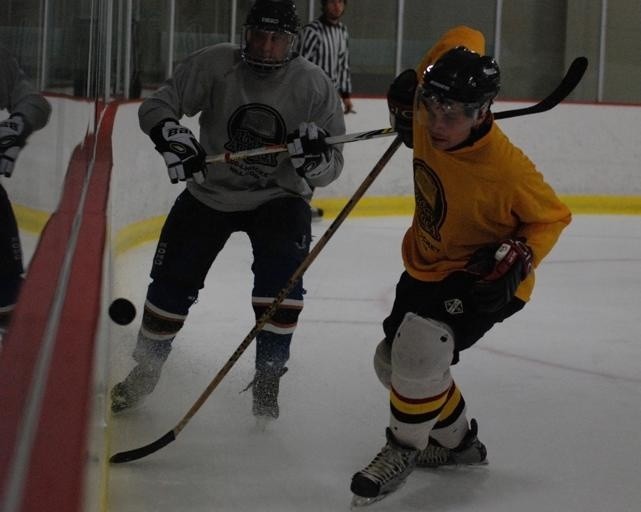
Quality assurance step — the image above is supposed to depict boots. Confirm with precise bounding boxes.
[350,419,487,498]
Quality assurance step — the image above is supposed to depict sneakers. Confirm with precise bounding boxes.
[113,362,160,408]
[251,366,290,419]
[312,204,323,225]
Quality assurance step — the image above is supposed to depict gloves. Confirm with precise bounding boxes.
[444,236,535,314]
[284,120,336,181]
[1,112,31,179]
[146,115,209,190]
[387,67,419,150]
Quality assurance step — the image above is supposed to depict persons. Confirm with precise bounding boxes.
[290,0,355,114]
[349,27,573,499]
[0,41,51,332]
[107,0,346,420]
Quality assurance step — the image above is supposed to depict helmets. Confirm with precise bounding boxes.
[417,45,501,131]
[240,1,303,69]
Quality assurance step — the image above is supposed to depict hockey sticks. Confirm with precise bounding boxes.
[207,58,588,162]
[109,132,402,463]
